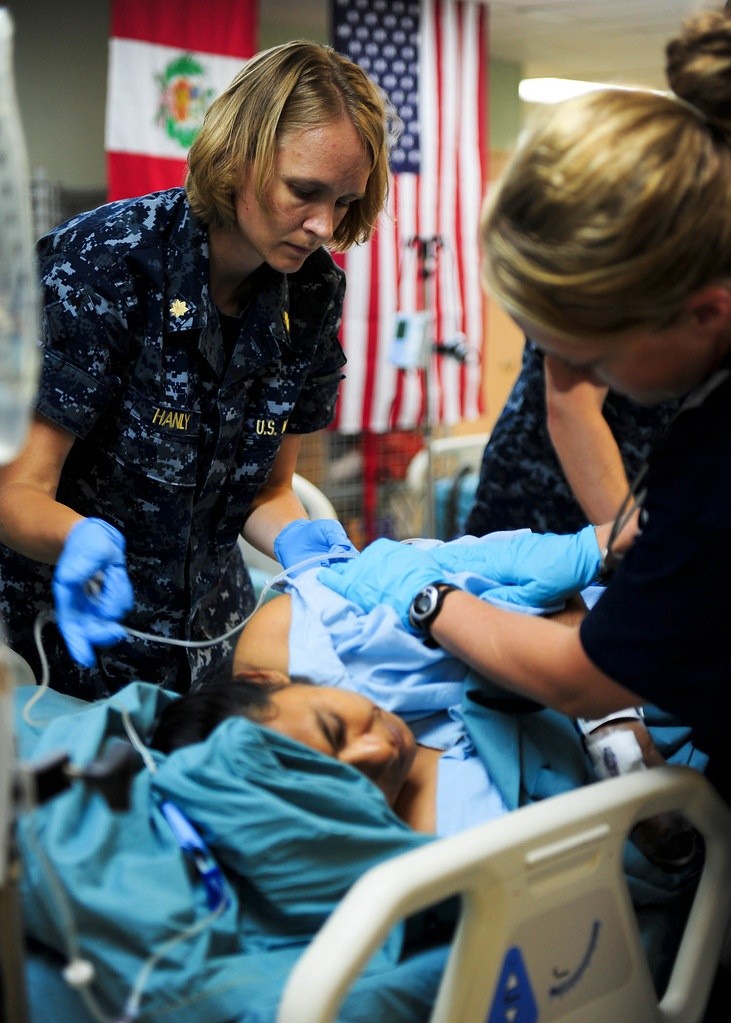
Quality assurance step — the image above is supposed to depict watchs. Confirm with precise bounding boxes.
[408,579,461,635]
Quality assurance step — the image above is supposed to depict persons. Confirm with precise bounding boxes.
[462,2,731,534]
[399,7,731,997]
[147,508,643,839]
[0,38,396,705]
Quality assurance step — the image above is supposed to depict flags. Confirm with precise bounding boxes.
[105,1,260,201]
[321,0,486,433]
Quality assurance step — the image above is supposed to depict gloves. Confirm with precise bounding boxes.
[274,518,353,572]
[315,538,443,636]
[430,525,602,609]
[54,515,133,668]
